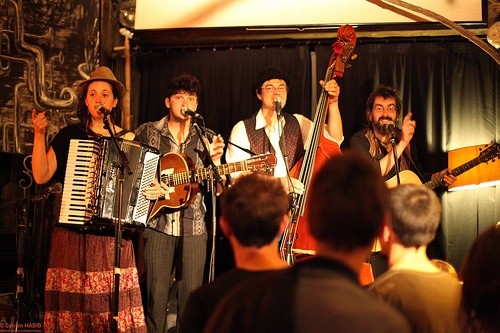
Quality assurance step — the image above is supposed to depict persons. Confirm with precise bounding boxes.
[350,85,457,280]
[180,172,289,333]
[456,225,500,333]
[133,73,225,333]
[225,68,344,196]
[31,66,161,333]
[206,151,407,333]
[367,182,469,333]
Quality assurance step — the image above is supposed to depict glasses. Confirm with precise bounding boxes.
[374,105,397,112]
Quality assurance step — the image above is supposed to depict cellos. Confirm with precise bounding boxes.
[272,24,358,267]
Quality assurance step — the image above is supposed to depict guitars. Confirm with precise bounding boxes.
[147,152,276,221]
[368,141,500,254]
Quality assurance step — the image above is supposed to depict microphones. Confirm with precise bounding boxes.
[386,121,397,141]
[96,104,112,115]
[274,97,281,116]
[182,107,202,118]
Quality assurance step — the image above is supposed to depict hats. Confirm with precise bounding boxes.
[77,65,125,102]
[255,65,293,91]
